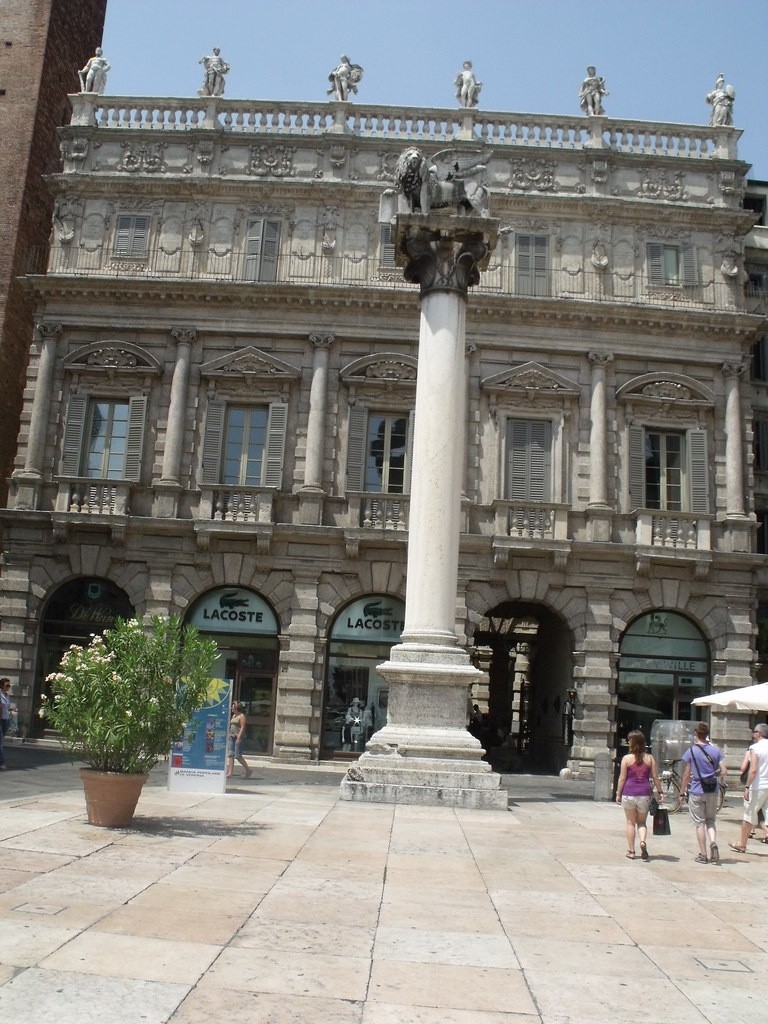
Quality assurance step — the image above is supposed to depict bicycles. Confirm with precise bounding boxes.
[647,759,724,814]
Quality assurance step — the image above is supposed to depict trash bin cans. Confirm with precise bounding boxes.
[594,751,613,801]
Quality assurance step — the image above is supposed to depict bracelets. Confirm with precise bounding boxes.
[680,795,685,797]
[746,787,749,788]
[657,792,663,794]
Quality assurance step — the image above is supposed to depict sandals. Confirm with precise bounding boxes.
[640,841,648,859]
[626,850,635,859]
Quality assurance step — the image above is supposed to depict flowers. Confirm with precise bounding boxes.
[37,612,223,774]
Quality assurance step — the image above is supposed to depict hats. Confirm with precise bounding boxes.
[350,698,361,705]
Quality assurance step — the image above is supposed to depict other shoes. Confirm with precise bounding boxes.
[695,853,708,864]
[226,774,234,778]
[728,843,746,853]
[748,833,756,839]
[710,842,719,862]
[244,769,253,780]
[761,837,768,844]
[1,765,7,769]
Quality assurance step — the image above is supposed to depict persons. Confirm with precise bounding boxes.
[728,723,768,852]
[202,47,229,96]
[679,722,727,864]
[454,60,481,108]
[329,55,355,100]
[615,730,664,859]
[345,697,362,752]
[80,47,110,92]
[227,701,252,779]
[707,74,734,126]
[578,65,607,116]
[0,678,12,769]
[473,704,482,722]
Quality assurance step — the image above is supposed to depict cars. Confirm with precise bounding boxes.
[332,706,348,715]
[326,706,332,713]
[325,711,345,731]
[240,700,272,749]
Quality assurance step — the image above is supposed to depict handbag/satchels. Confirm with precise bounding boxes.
[653,804,671,835]
[649,798,659,816]
[700,777,717,793]
[740,762,751,785]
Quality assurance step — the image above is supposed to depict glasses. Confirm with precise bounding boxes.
[752,730,759,734]
[4,685,11,687]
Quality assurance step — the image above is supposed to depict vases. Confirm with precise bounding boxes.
[78,768,148,827]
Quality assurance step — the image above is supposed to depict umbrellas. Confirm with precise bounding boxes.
[692,682,768,714]
